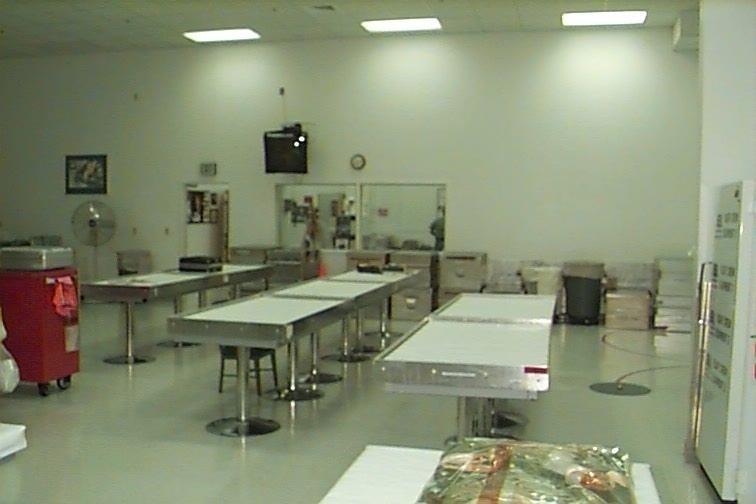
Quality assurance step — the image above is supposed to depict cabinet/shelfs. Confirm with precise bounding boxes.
[0,268,80,396]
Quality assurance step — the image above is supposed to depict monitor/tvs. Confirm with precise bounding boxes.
[263,131,308,172]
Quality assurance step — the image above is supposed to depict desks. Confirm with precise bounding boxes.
[317,441,662,504]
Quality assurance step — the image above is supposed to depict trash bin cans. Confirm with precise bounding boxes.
[563,260,603,324]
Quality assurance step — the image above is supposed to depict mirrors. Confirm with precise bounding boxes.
[180,178,229,260]
[273,182,449,253]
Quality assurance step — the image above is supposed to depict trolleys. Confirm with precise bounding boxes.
[0,240,86,400]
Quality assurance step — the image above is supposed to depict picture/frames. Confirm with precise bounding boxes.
[64,153,108,194]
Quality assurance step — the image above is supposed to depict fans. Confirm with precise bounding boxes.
[70,199,118,248]
